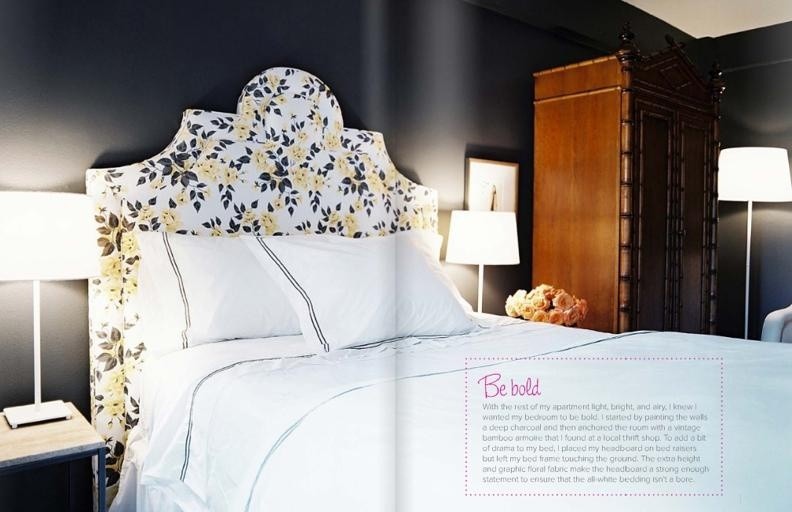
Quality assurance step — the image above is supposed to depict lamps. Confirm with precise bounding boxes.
[443,209,522,313]
[0,189,104,430]
[717,146,792,342]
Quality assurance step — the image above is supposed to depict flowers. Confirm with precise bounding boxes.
[504,283,591,327]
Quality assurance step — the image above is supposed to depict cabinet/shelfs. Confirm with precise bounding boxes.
[530,29,728,335]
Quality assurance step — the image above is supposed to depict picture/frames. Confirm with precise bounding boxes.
[464,156,519,219]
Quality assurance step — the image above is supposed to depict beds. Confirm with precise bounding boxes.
[85,63,791,512]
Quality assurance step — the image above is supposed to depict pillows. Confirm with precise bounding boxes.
[240,228,492,362]
[132,226,302,361]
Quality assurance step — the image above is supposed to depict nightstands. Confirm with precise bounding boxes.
[0,400,111,511]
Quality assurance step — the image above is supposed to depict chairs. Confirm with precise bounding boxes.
[759,304,792,344]
